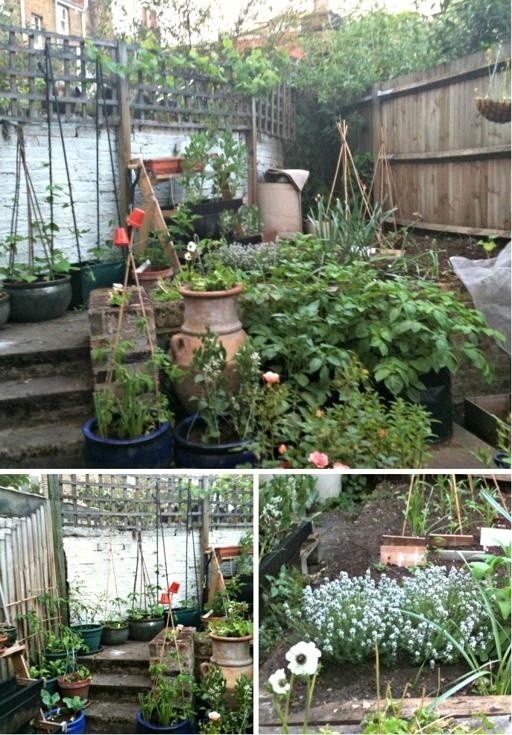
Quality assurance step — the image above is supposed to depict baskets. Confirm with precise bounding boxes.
[476,98,511,123]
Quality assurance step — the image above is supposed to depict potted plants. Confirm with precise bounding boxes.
[169,239,248,415]
[58,665,91,709]
[359,277,506,446]
[200,601,253,689]
[42,163,127,311]
[17,592,90,668]
[175,325,263,469]
[179,128,249,241]
[0,222,81,323]
[69,571,111,655]
[162,584,199,631]
[135,683,197,734]
[99,596,130,645]
[29,659,68,696]
[127,584,165,641]
[82,292,192,469]
[259,474,319,588]
[132,203,204,293]
[41,689,88,734]
